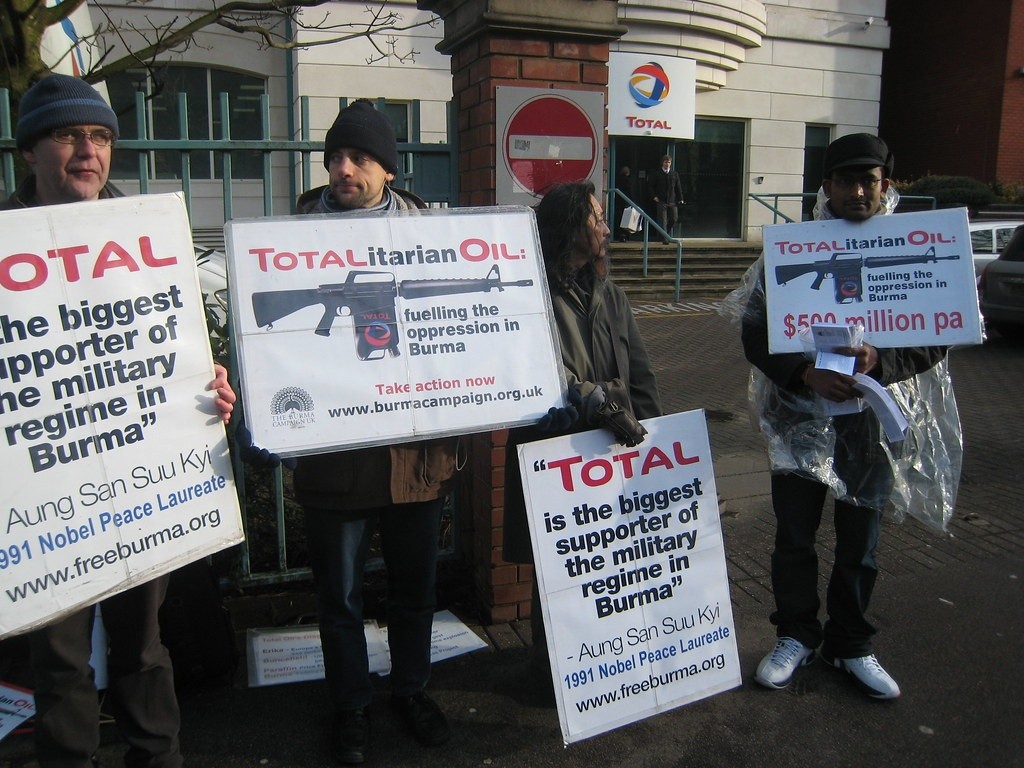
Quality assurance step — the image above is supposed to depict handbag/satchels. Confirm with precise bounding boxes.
[620,206,643,234]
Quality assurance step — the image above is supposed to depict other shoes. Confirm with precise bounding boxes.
[663,241,668,245]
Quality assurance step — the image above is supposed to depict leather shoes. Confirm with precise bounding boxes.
[331,702,373,763]
[392,689,451,746]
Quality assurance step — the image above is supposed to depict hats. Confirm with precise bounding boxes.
[16,74,120,145]
[324,97,399,174]
[823,133,893,181]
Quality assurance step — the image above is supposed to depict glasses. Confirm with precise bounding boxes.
[47,126,117,147]
[833,173,884,190]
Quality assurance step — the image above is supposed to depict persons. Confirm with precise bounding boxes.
[0,73,235,768]
[740,133,950,699]
[236,100,455,768]
[501,181,661,694]
[614,162,641,243]
[647,155,684,244]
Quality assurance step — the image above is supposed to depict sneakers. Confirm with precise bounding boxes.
[754,637,816,689]
[820,649,901,699]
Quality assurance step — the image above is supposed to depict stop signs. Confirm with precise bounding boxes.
[495,85,604,210]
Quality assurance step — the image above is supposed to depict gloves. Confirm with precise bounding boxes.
[234,420,300,473]
[538,406,579,430]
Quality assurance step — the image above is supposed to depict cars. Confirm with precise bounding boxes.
[193,241,239,352]
[976,223,1024,339]
[969,218,1024,289]
